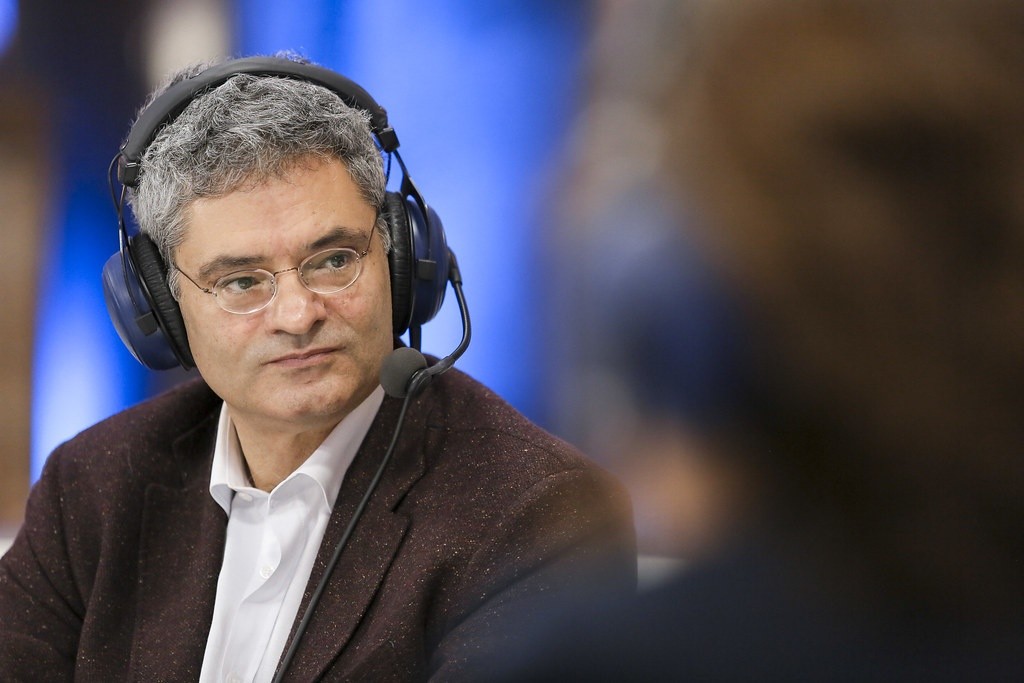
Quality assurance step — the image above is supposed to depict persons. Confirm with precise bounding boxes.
[0,54,645,683]
[433,0,1024,683]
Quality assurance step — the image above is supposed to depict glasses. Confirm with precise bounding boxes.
[170,207,380,314]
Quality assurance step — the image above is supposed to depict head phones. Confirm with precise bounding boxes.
[100,55,451,372]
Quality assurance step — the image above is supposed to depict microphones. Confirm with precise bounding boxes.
[378,246,471,400]
